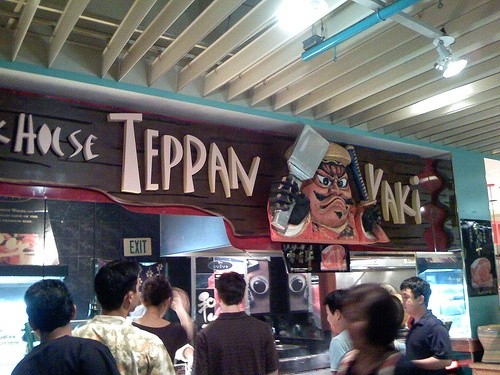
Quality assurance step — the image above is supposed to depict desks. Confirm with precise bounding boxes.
[469,363,500,375]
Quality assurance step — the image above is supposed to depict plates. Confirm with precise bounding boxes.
[247,260,258,269]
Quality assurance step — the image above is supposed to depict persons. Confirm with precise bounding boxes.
[324,289,355,375]
[335,283,426,375]
[400,277,454,375]
[190,272,279,375]
[70,258,195,375]
[11,279,121,375]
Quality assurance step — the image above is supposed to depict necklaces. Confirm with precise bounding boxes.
[49,335,65,339]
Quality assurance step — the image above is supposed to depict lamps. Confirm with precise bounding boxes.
[433,36,467,78]
[302,34,325,51]
